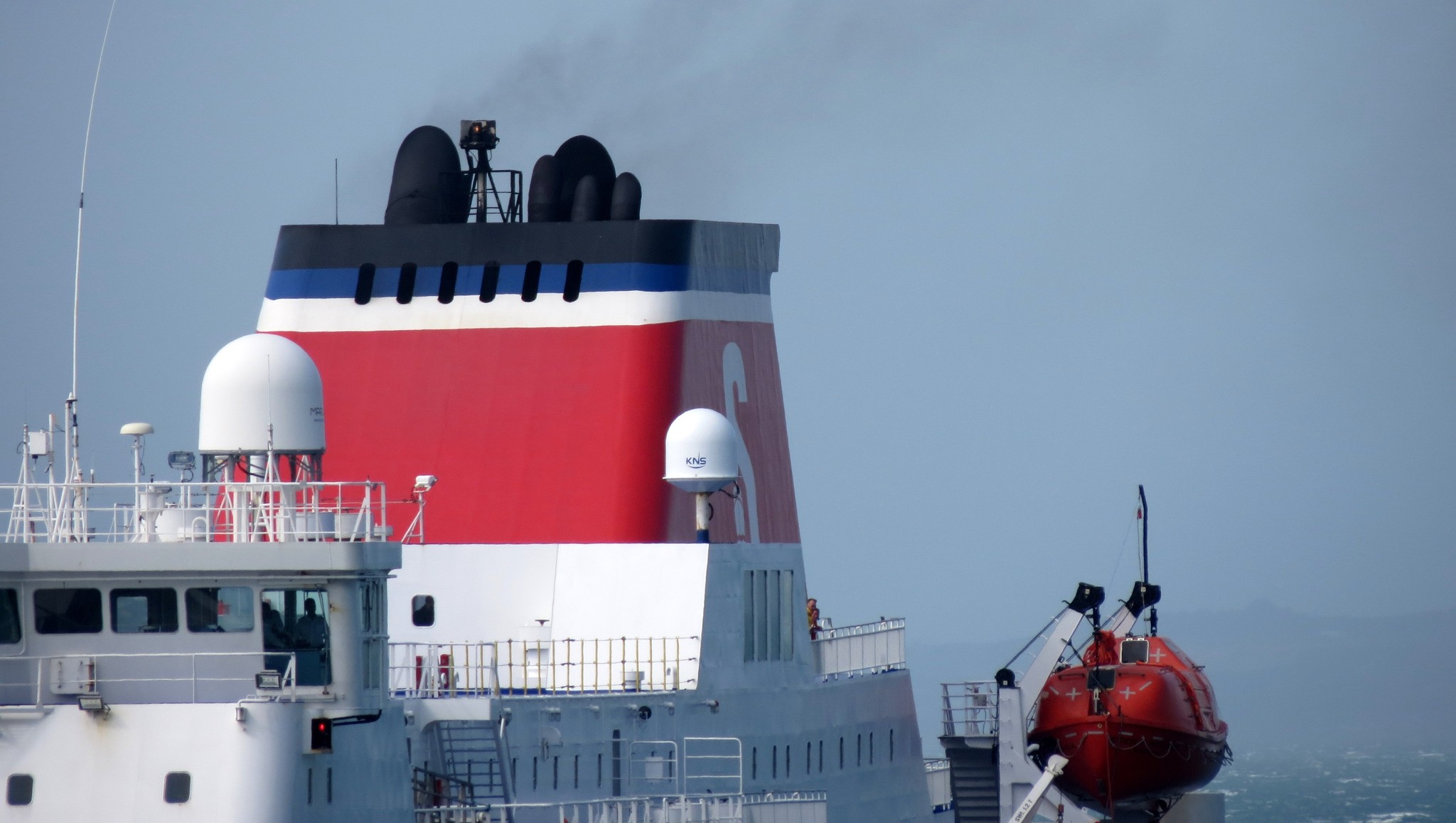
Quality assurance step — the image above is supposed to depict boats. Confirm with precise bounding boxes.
[1,119,1231,823]
[1024,629,1230,823]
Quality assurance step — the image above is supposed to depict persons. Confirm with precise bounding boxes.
[260,598,333,659]
[807,599,824,640]
